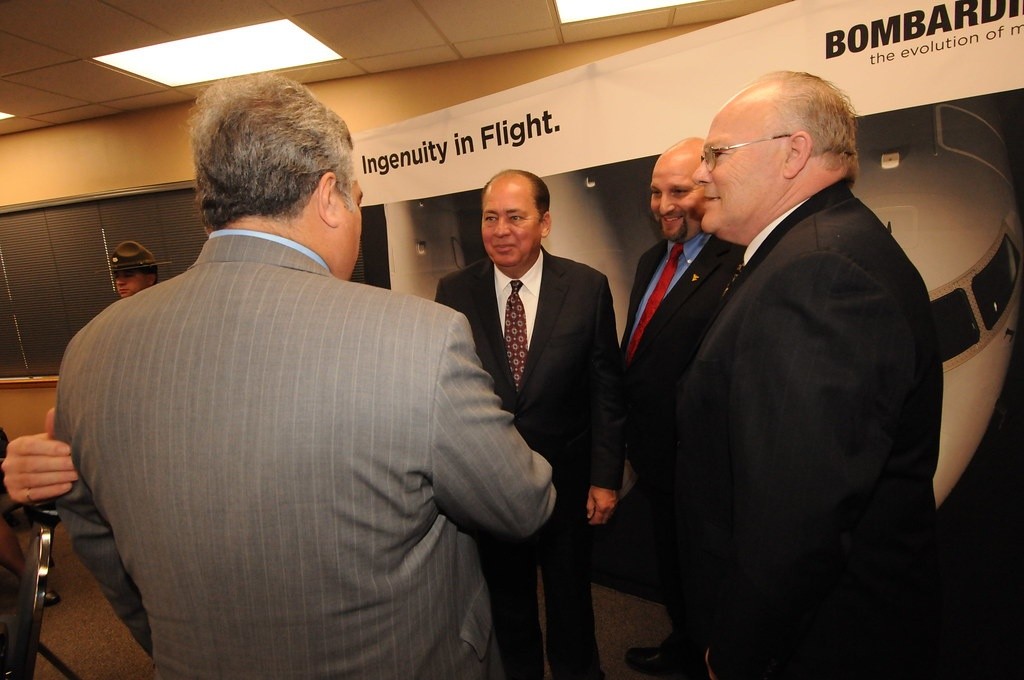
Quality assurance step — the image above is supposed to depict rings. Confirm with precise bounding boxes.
[27,488,32,502]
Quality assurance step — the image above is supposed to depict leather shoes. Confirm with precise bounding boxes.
[624,647,673,674]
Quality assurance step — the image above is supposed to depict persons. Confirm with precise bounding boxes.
[673,71,944,680]
[1,407,78,503]
[96,241,172,298]
[619,138,749,680]
[53,74,556,680]
[434,171,626,680]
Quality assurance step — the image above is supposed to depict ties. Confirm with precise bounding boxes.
[504,280,528,393]
[719,263,744,302]
[625,242,683,368]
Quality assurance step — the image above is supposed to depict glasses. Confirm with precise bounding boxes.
[701,134,792,173]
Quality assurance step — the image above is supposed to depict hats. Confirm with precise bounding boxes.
[97,241,172,274]
